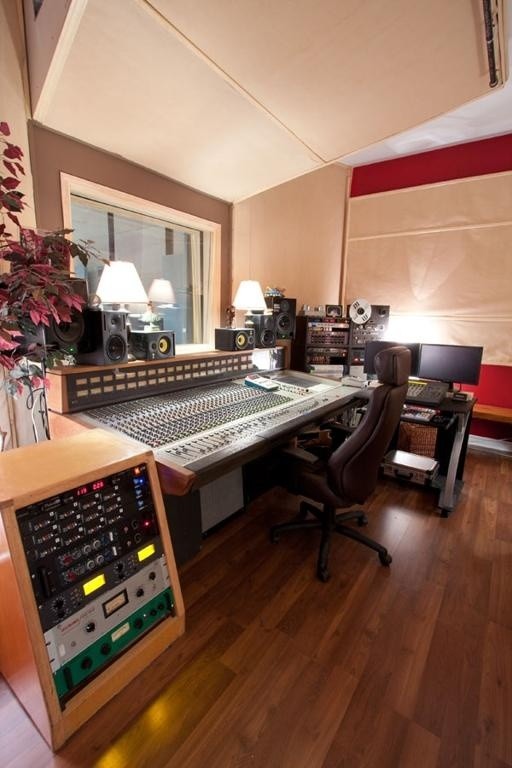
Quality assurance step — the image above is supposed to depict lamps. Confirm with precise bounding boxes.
[149,279,175,304]
[92,261,150,362]
[232,280,267,329]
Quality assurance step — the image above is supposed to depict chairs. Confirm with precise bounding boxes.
[265,346,412,581]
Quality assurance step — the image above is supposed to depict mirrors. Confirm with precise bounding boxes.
[60,171,222,355]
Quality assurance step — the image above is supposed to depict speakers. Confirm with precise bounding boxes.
[82,310,129,366]
[264,297,297,340]
[127,330,176,362]
[215,327,255,351]
[244,314,275,348]
[20,278,88,355]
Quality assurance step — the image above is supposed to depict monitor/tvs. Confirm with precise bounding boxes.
[364,340,420,388]
[418,343,483,393]
